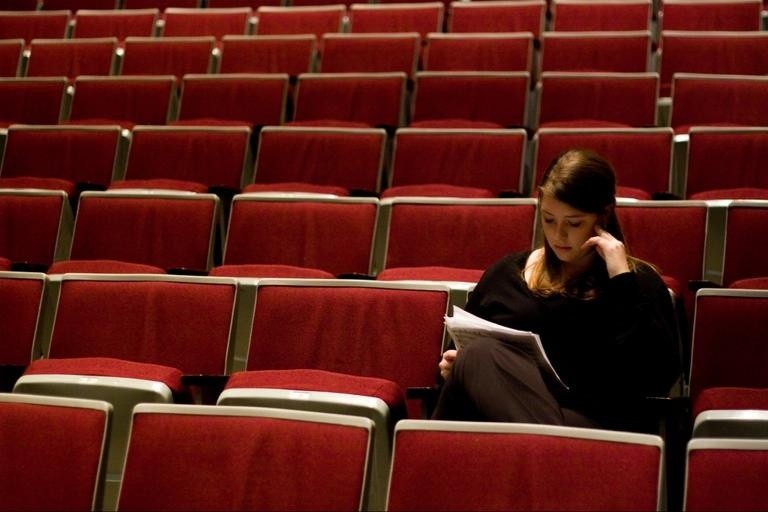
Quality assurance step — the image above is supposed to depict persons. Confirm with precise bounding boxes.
[424,151,683,438]
